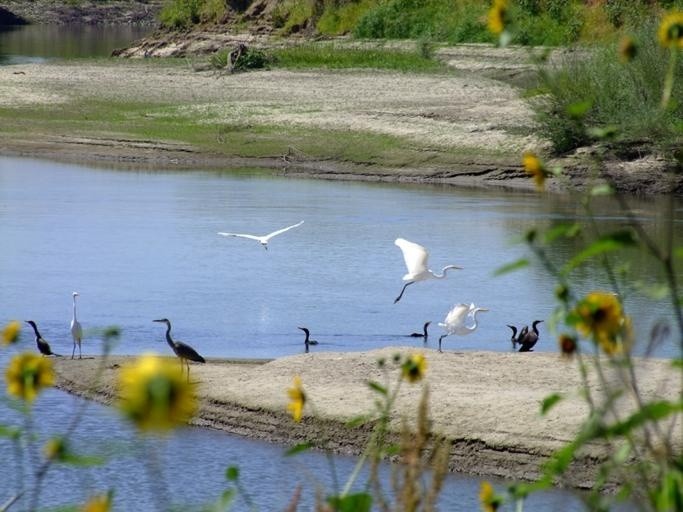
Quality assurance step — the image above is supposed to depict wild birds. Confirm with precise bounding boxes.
[409,320,431,338]
[296,326,317,346]
[69,292,82,360]
[437,301,489,353]
[506,319,545,352]
[152,316,207,385]
[216,221,306,252]
[24,319,53,357]
[392,237,463,305]
